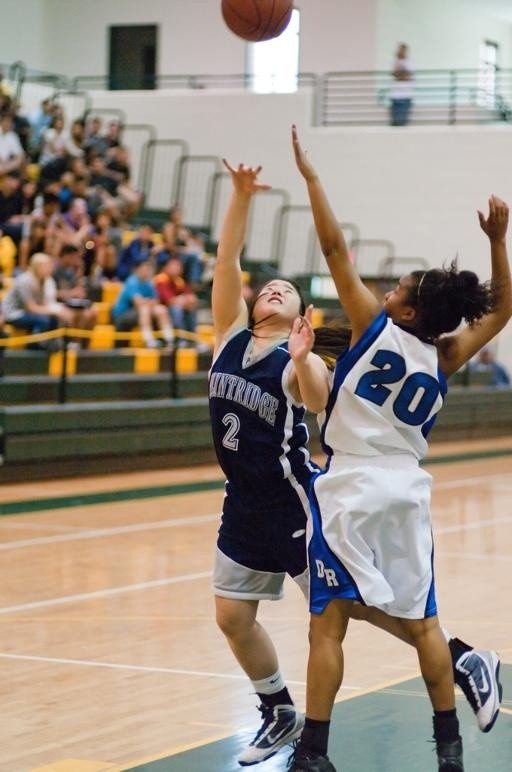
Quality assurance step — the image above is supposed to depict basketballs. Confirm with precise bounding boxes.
[220,1,293,40]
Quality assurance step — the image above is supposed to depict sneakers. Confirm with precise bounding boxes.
[286,742,337,772]
[145,340,210,358]
[236,704,305,768]
[436,737,464,772]
[453,646,503,733]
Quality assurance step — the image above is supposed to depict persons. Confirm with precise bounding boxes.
[0,76,255,355]
[389,42,416,127]
[200,155,505,765]
[281,118,512,772]
